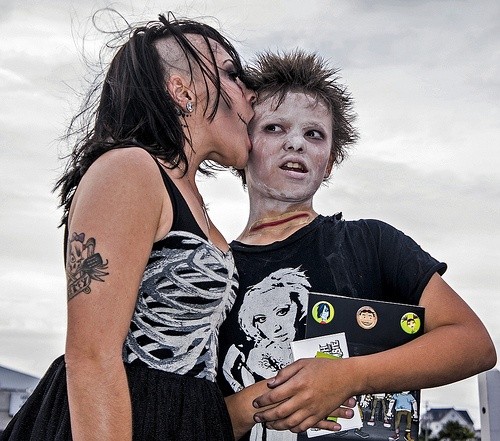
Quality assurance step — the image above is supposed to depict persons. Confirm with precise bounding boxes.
[2,13,258,441]
[220,50,497,440]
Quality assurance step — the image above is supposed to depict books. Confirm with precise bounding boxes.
[297,292,425,440]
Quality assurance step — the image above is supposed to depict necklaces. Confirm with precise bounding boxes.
[172,152,213,237]
